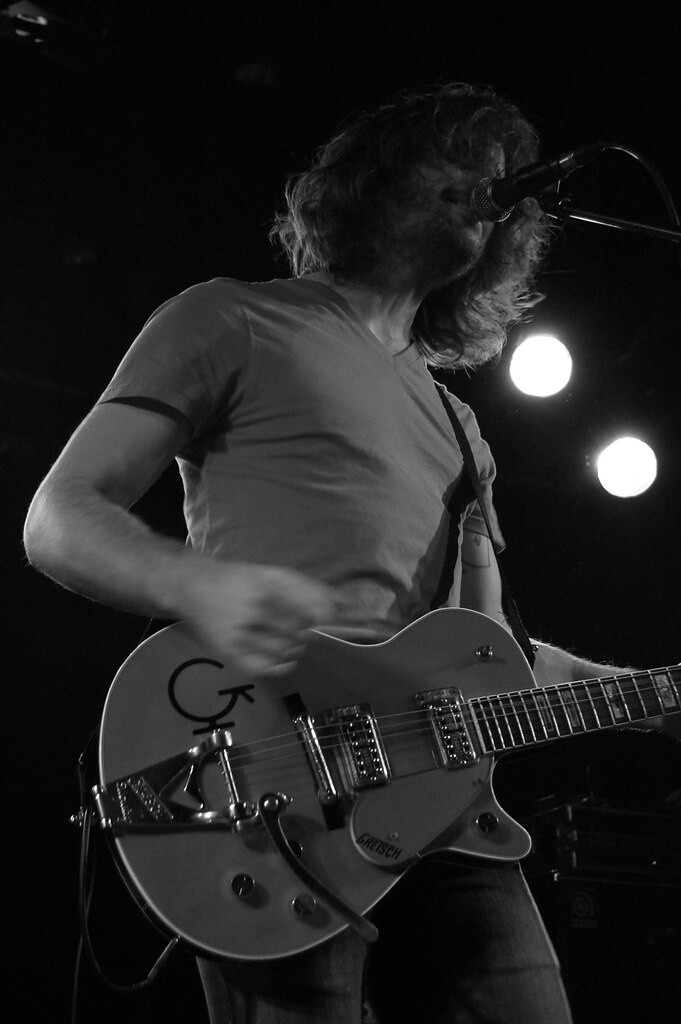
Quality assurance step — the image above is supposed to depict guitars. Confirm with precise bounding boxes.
[96,600,680,980]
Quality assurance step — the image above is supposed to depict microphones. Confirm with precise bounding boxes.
[471,139,606,222]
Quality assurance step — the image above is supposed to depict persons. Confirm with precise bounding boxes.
[22,84,671,1024]
